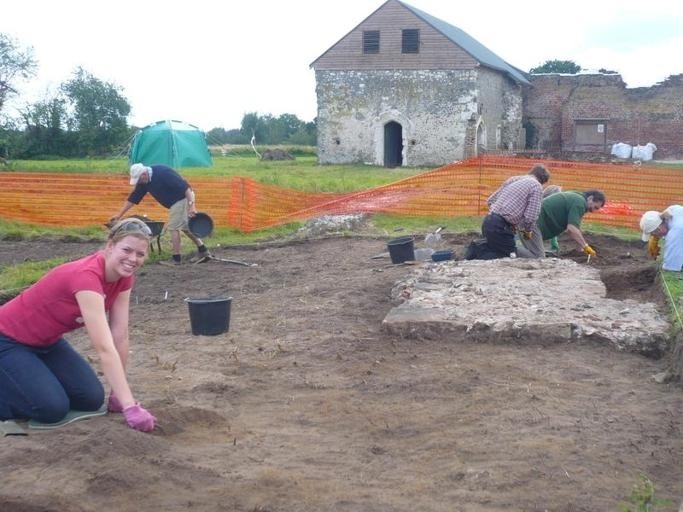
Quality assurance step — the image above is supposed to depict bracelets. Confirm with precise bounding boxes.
[581,243,589,249]
[188,201,195,206]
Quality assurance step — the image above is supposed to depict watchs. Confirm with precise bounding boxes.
[107,162,214,266]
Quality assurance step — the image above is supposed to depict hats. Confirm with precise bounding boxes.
[129,162,144,185]
[639,210,663,242]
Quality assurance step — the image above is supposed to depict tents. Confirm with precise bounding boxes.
[128,119,213,172]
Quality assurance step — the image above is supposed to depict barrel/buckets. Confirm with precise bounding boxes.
[188,213,214,237]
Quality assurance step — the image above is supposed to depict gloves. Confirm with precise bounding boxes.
[583,246,597,257]
[551,236,559,250]
[108,389,124,413]
[648,236,661,256]
[123,400,157,432]
[522,230,532,240]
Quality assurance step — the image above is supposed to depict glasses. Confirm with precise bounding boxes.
[113,220,154,237]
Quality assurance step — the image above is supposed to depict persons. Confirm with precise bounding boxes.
[512,184,563,256]
[464,163,551,260]
[0,218,158,435]
[512,189,607,260]
[639,203,683,271]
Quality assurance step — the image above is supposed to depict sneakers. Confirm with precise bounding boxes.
[159,258,182,268]
[190,249,212,264]
